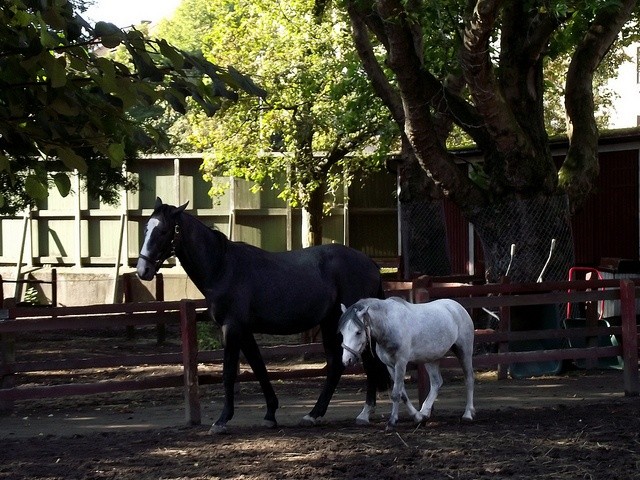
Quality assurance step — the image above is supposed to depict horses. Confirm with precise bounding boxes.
[136,196,385,436]
[338,297,475,430]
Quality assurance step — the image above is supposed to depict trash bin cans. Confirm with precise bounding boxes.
[598,257,638,319]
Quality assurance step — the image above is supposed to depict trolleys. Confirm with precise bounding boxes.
[565,266,626,372]
[486,239,562,379]
